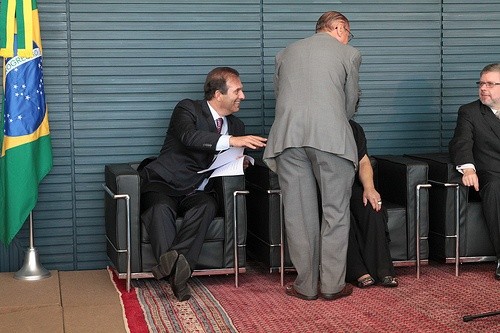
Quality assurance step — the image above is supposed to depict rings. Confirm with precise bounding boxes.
[378,200,383,205]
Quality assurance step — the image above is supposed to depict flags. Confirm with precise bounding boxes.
[0,0,54,247]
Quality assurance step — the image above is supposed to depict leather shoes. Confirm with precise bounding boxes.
[152,249,191,302]
[495,259,500,279]
[322,283,354,300]
[285,285,319,301]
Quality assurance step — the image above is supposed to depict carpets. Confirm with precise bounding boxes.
[106,263,500,333]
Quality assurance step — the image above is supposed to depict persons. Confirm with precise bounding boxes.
[140,67,268,302]
[349,87,399,287]
[263,10,363,301]
[448,64,500,281]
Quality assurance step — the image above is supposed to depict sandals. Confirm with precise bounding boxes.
[381,274,399,287]
[358,272,376,288]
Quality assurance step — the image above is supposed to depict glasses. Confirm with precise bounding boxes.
[333,23,354,42]
[476,82,500,89]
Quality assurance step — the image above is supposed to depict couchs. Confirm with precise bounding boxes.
[103,152,500,292]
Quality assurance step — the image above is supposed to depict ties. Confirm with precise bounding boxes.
[216,117,224,135]
[496,110,500,117]
